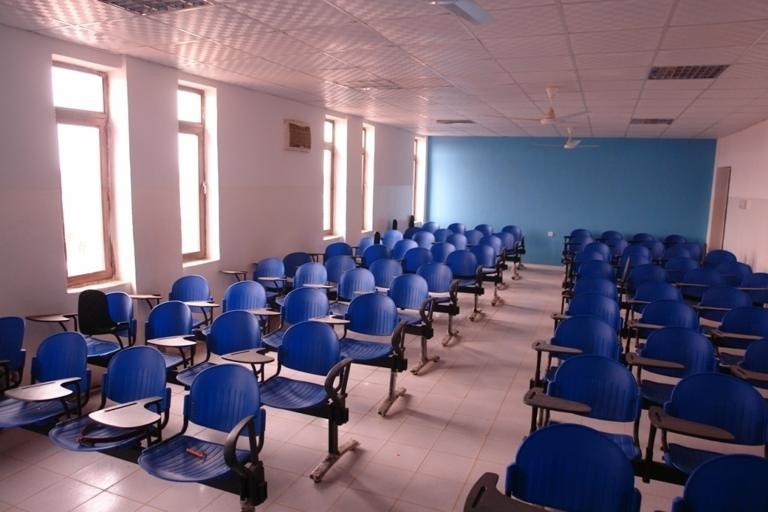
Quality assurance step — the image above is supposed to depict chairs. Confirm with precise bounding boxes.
[493,231,520,279]
[323,242,353,265]
[411,230,435,254]
[390,240,419,259]
[357,237,383,256]
[629,264,669,295]
[741,342,767,385]
[326,256,358,286]
[702,250,737,267]
[402,247,432,272]
[638,241,666,262]
[665,257,699,273]
[1,331,91,428]
[567,293,619,329]
[478,236,508,290]
[78,290,133,353]
[685,242,702,261]
[143,300,196,375]
[252,258,286,303]
[380,229,403,253]
[635,299,698,350]
[661,373,767,480]
[670,454,766,512]
[573,250,605,272]
[0,316,28,398]
[712,261,752,283]
[47,347,171,452]
[259,322,360,483]
[329,267,377,316]
[199,279,266,340]
[446,250,484,322]
[422,222,436,232]
[167,273,214,332]
[700,286,754,327]
[632,282,683,312]
[70,291,136,362]
[741,273,768,307]
[638,327,719,405]
[275,263,328,308]
[503,423,642,512]
[261,288,331,351]
[545,317,622,389]
[447,234,467,251]
[435,229,453,242]
[465,230,483,247]
[720,310,768,350]
[448,223,466,235]
[631,233,653,244]
[575,262,614,283]
[683,269,723,297]
[475,224,491,237]
[570,237,595,251]
[544,357,641,461]
[599,230,625,239]
[664,247,691,261]
[622,245,652,262]
[471,245,502,306]
[386,273,441,376]
[283,252,311,282]
[573,278,618,303]
[403,227,419,238]
[339,293,407,418]
[617,253,650,275]
[137,364,268,512]
[369,258,403,292]
[501,226,525,270]
[415,261,460,346]
[570,229,594,243]
[597,239,630,257]
[430,242,456,263]
[363,245,388,266]
[584,243,613,262]
[175,311,262,390]
[664,234,689,245]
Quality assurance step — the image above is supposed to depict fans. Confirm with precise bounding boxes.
[534,127,600,149]
[486,88,592,125]
